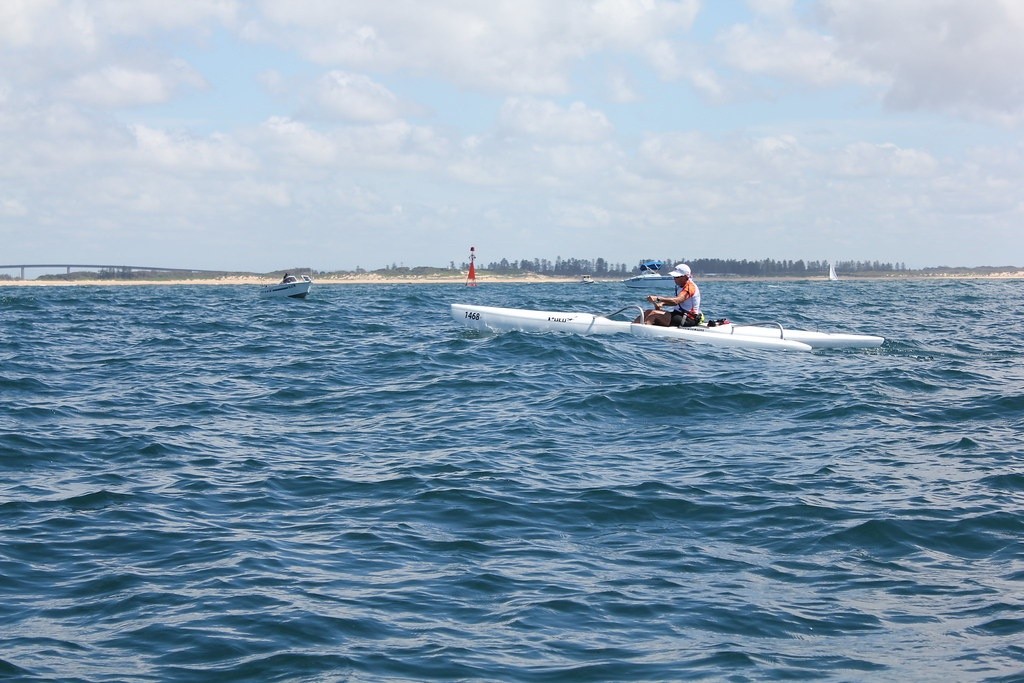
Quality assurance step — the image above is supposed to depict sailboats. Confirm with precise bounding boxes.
[829,263,839,281]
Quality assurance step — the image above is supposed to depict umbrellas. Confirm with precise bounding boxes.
[640,260,663,274]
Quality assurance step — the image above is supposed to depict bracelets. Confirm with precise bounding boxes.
[658,296,662,302]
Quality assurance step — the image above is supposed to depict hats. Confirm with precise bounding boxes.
[667,264,691,277]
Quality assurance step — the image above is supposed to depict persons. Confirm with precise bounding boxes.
[633,263,701,327]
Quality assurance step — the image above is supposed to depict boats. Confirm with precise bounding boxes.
[580,275,594,285]
[259,275,312,299]
[451,303,884,353]
[621,261,678,290]
